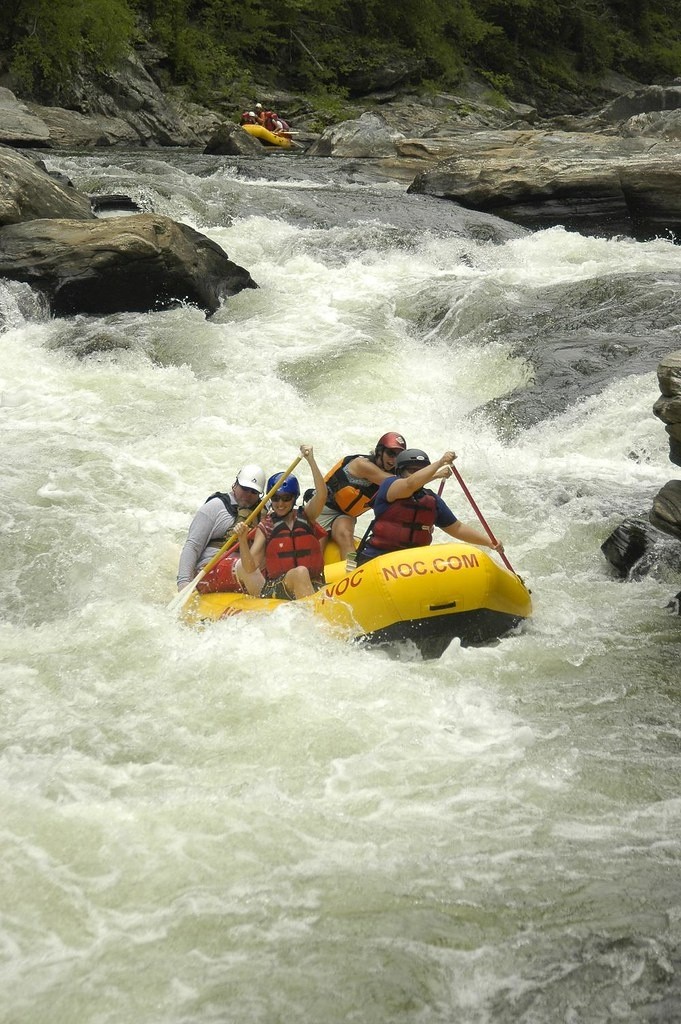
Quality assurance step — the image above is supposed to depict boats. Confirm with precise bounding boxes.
[242,123,292,148]
[174,540,533,658]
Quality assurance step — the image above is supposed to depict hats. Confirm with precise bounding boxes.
[236,464,266,494]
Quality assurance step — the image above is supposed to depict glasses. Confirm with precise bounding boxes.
[404,468,420,474]
[271,495,294,502]
[385,449,399,458]
[240,485,258,494]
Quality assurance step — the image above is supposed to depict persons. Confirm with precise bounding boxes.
[233,445,329,601]
[354,449,505,568]
[243,103,289,132]
[177,465,270,595]
[308,432,452,562]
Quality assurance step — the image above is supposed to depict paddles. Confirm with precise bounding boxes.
[290,139,305,148]
[275,132,299,135]
[437,462,516,574]
[165,450,308,619]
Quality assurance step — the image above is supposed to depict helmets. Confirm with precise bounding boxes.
[396,448,431,467]
[249,111,255,116]
[270,113,278,119]
[378,431,406,450]
[266,472,300,499]
[255,103,262,109]
[266,112,272,118]
